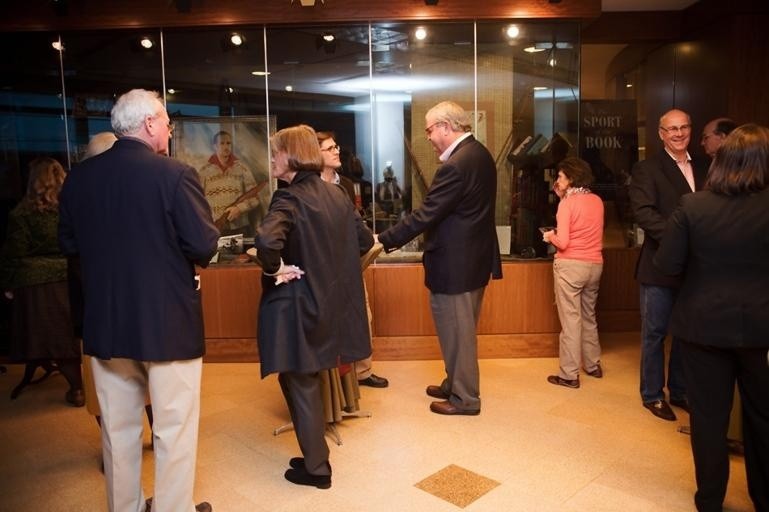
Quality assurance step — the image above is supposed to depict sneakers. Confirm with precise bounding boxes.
[591,367,604,378]
[547,374,579,388]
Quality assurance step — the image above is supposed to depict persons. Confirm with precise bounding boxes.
[654,125,769,511]
[627,110,744,420]
[543,158,604,388]
[252,123,375,490]
[55,87,215,511]
[372,100,504,414]
[312,131,403,423]
[7,132,119,404]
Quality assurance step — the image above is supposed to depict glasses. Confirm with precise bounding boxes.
[424,122,444,133]
[322,146,340,153]
[662,125,689,134]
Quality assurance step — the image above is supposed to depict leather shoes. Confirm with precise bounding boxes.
[290,458,331,472]
[284,469,330,489]
[431,400,481,415]
[359,375,388,388]
[427,385,449,399]
[644,400,675,420]
[64,389,84,405]
